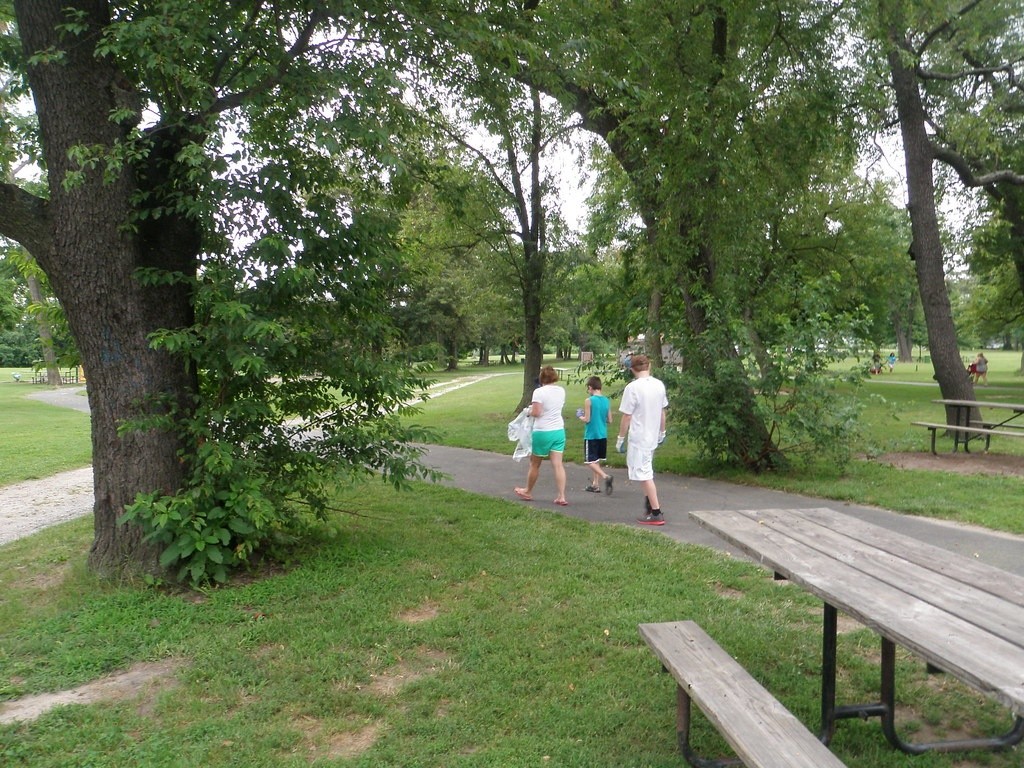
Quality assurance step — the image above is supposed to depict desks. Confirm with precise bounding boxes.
[929,398,1024,454]
[688,505,1024,753]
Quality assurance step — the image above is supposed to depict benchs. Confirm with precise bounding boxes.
[910,420,1023,455]
[638,616,850,768]
[966,418,1024,451]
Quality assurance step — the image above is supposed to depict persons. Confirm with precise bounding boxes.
[973,351,989,387]
[614,354,670,526]
[872,350,883,375]
[625,350,634,369]
[887,352,897,372]
[510,363,568,505]
[576,375,615,496]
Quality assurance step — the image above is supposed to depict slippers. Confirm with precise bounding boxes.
[552,499,568,505]
[513,487,533,500]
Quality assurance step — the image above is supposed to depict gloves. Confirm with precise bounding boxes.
[576,409,583,417]
[615,436,625,453]
[658,430,666,443]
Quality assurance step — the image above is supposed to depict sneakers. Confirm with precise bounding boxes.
[585,485,599,493]
[605,475,613,495]
[635,512,665,525]
